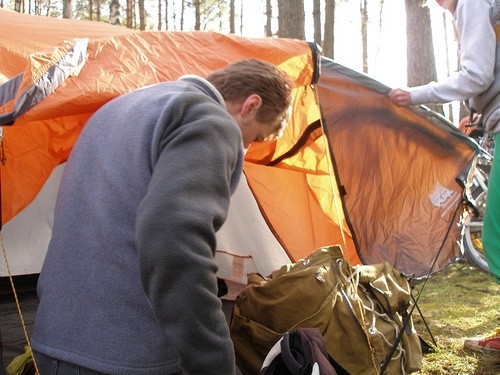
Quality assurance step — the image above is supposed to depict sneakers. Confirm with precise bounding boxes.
[464,327,500,354]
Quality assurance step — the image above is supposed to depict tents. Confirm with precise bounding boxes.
[316,54,494,276]
[3,7,368,375]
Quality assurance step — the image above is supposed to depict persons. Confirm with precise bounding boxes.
[388,0,499,356]
[29,60,296,375]
